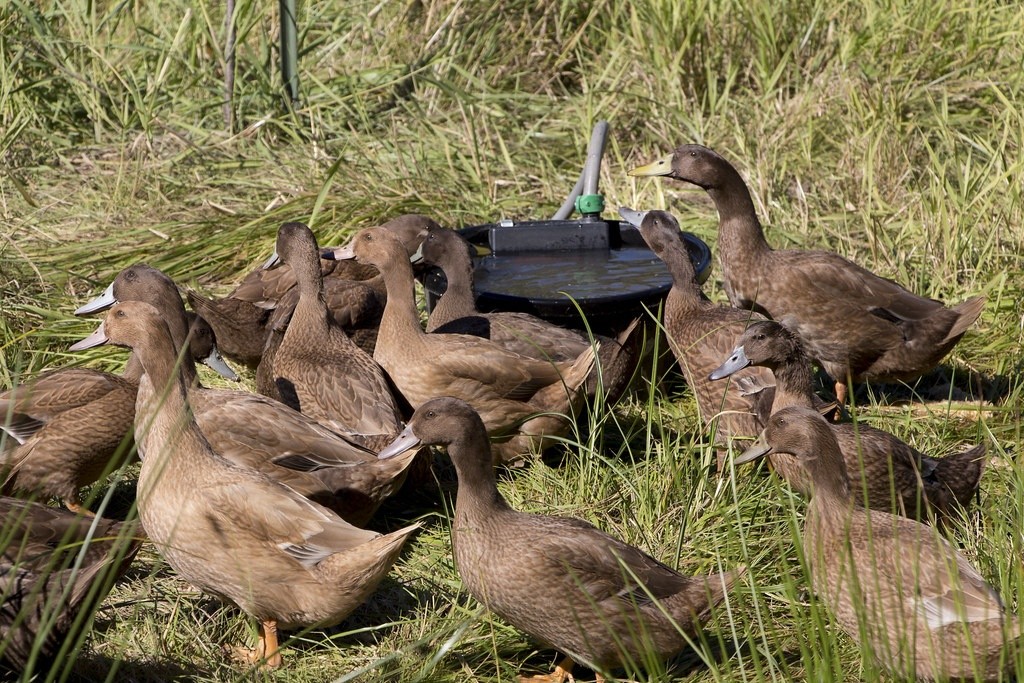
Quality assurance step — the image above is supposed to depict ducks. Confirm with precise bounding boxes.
[625,142,988,419]
[619,204,989,541]
[733,406,1024,683]
[0,214,643,676]
[377,394,751,683]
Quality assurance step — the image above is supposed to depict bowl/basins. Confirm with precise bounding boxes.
[413,221,715,378]
